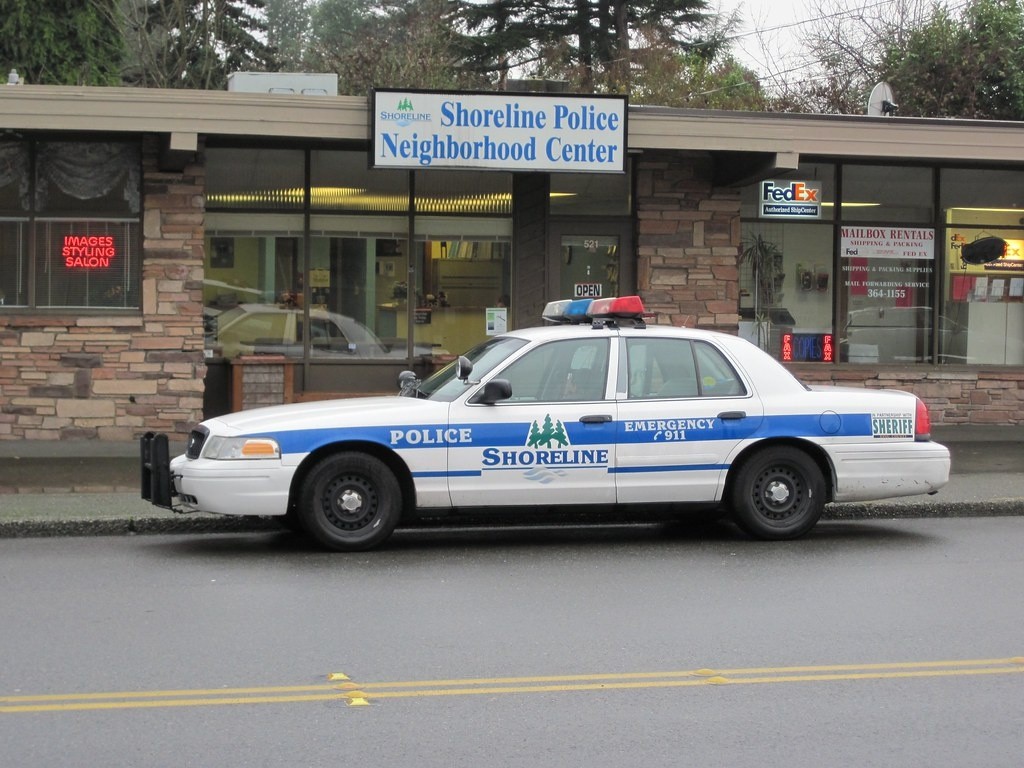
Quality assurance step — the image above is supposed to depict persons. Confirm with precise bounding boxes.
[496,295,510,307]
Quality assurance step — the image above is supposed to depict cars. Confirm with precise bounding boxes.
[203,292,452,358]
[141,293,952,553]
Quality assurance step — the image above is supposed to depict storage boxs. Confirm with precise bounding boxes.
[840,343,879,362]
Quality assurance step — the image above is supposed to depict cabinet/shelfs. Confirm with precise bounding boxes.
[423,241,505,307]
[943,300,1024,364]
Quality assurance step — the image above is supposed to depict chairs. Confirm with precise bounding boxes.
[563,367,591,398]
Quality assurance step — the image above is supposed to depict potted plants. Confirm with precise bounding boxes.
[388,280,407,306]
[738,231,776,288]
